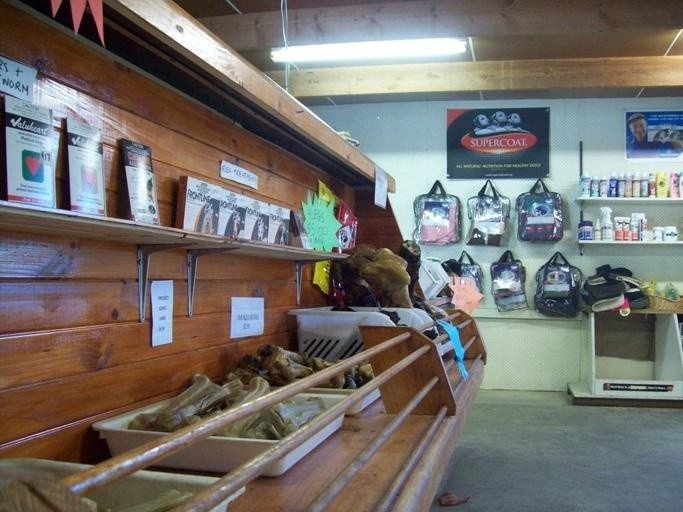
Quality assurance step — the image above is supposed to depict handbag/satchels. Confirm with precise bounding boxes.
[441,251,482,293]
[534,252,583,317]
[465,180,510,246]
[490,251,529,312]
[413,180,461,246]
[516,178,563,241]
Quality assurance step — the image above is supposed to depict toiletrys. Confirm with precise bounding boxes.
[594,218,601,239]
[629,214,638,240]
[614,216,622,240]
[617,173,625,197]
[580,177,591,198]
[578,222,593,240]
[638,213,647,242]
[669,171,679,198]
[591,177,599,197]
[621,217,631,240]
[656,171,668,197]
[609,173,617,196]
[678,172,683,199]
[648,172,656,197]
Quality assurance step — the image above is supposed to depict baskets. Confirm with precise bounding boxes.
[287,306,424,363]
[644,293,683,311]
[410,304,454,357]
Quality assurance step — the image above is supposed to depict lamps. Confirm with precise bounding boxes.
[270,37,468,62]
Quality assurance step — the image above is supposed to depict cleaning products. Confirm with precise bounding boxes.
[600,206,612,239]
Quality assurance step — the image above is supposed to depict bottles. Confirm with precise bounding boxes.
[618,172,626,198]
[649,173,656,197]
[594,219,602,240]
[610,172,618,197]
[664,226,678,241]
[622,221,630,240]
[591,176,600,197]
[615,221,622,240]
[639,213,645,241]
[630,213,639,240]
[579,172,590,198]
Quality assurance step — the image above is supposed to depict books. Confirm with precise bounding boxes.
[2,94,57,210]
[66,116,107,217]
[120,137,161,227]
[175,176,315,252]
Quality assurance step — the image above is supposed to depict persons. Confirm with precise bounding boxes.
[627,114,647,152]
[470,111,532,136]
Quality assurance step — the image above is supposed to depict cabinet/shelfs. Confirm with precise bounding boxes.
[573,198,683,256]
[0,0,490,512]
